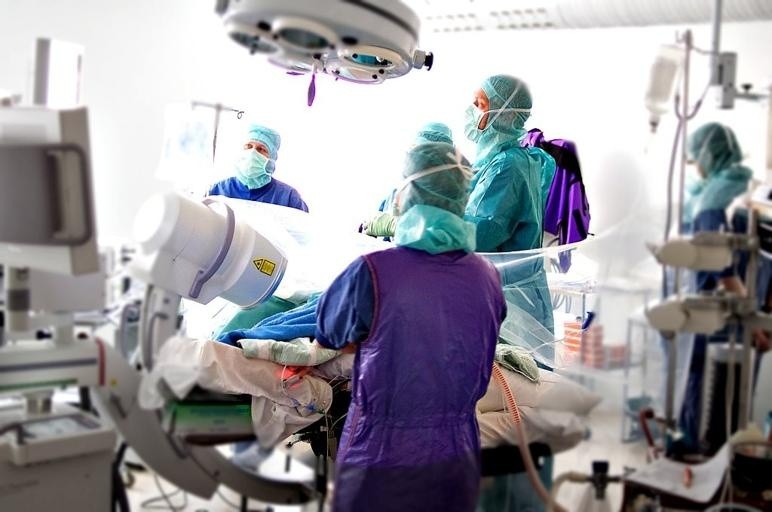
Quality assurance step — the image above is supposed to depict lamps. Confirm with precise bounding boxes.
[215,0,435,87]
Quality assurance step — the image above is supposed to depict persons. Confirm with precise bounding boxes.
[676,121,772,459]
[377,123,455,241]
[357,74,552,511]
[204,123,311,472]
[313,141,509,510]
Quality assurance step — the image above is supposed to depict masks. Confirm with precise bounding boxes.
[237,149,272,178]
[463,103,485,143]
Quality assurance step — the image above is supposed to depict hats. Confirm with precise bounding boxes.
[476,75,533,149]
[400,140,473,221]
[419,122,453,145]
[234,124,281,189]
[688,121,742,177]
